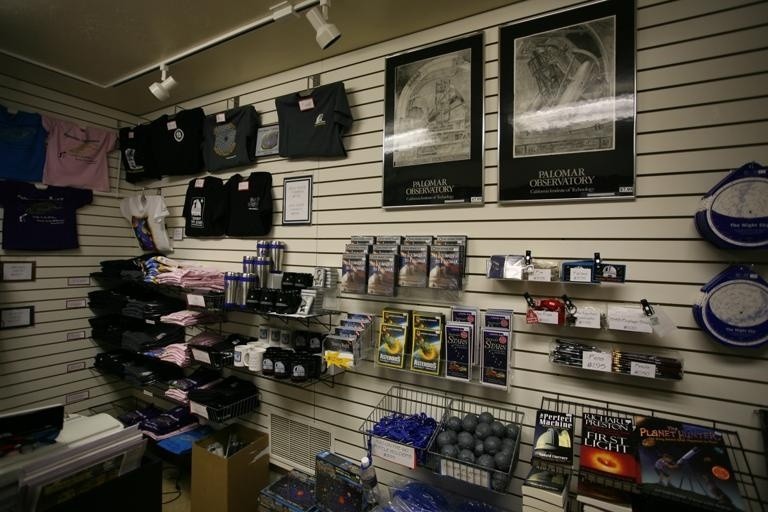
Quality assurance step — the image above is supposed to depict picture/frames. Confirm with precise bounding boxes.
[0,261,36,282]
[0,306,34,329]
[282,175,312,224]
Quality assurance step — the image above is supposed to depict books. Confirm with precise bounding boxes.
[520,407,747,511]
[322,233,515,391]
[23,423,150,512]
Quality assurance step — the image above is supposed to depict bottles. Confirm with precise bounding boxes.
[358,457,381,505]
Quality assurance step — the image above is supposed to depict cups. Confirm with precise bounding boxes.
[222,256,313,314]
[256,239,270,257]
[232,323,326,384]
[268,241,285,274]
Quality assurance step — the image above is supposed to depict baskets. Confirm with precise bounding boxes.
[179,292,227,315]
[357,387,524,496]
[183,342,232,370]
[188,385,261,425]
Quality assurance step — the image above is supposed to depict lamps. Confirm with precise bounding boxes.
[148,63,177,102]
[306,0,342,50]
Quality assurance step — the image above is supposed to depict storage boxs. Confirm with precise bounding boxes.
[191,423,269,512]
